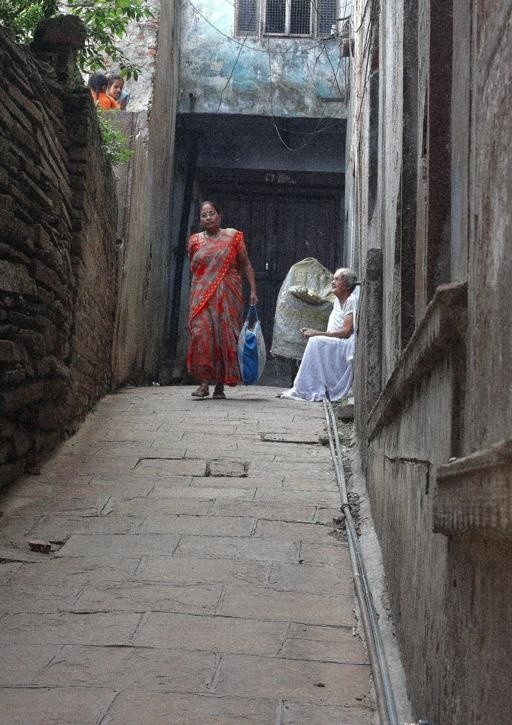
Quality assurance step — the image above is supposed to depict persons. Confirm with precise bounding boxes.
[276,266,359,404]
[108,74,131,111]
[88,74,121,110]
[187,199,258,400]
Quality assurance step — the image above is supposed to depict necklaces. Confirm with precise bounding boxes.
[204,228,222,240]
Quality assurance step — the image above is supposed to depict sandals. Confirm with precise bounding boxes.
[191,388,225,399]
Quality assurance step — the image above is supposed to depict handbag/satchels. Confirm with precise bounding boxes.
[237,304,266,385]
